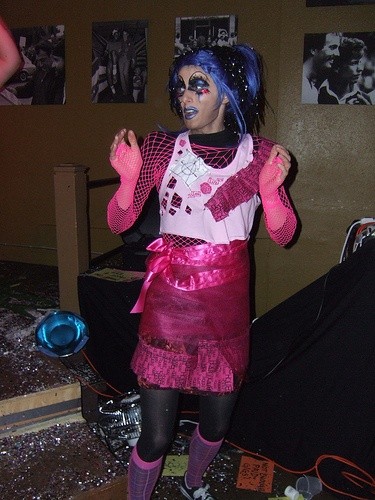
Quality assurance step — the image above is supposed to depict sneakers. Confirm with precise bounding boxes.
[178,470,217,500]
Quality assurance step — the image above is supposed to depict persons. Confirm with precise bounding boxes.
[5,22,375,105]
[107,43,298,500]
[0,20,22,93]
